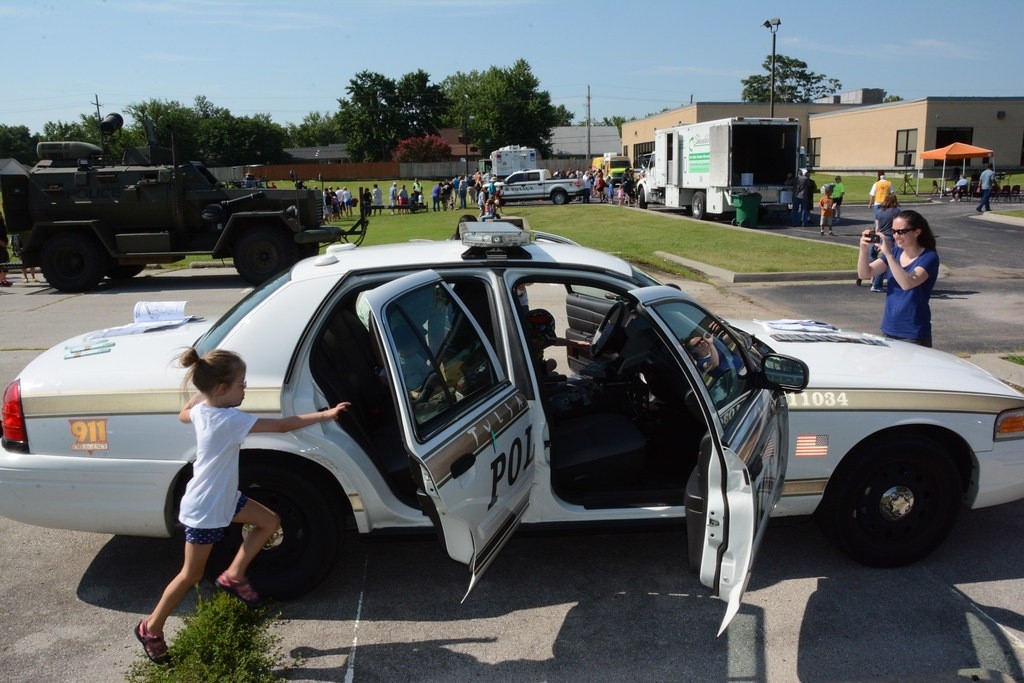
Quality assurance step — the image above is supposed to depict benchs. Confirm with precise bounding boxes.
[373,204,422,213]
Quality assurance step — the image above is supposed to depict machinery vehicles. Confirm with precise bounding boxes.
[1,96,369,293]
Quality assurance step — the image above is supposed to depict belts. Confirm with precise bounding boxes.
[886,234,892,236]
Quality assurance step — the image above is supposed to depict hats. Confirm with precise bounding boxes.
[490,177,497,181]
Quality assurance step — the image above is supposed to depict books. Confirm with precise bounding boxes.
[89,300,205,336]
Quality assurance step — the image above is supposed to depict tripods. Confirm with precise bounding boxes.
[894,167,916,196]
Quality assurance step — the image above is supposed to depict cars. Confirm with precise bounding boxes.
[1,206,1023,639]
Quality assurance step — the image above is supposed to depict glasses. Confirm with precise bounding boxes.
[691,337,705,350]
[891,225,919,236]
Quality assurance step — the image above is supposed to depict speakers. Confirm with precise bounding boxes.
[904,153,912,167]
[983,157,989,165]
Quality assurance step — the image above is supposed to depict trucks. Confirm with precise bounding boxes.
[490,144,536,185]
[636,115,805,221]
[591,151,633,188]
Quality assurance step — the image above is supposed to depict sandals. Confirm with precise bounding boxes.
[215,571,259,604]
[135,619,171,665]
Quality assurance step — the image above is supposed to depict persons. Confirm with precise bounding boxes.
[686,321,748,381]
[269,181,276,189]
[390,326,429,395]
[134,346,351,670]
[869,193,900,293]
[831,175,846,223]
[857,211,940,346]
[819,186,835,236]
[450,214,478,239]
[552,167,635,207]
[975,163,995,211]
[431,169,504,222]
[870,171,892,230]
[312,178,424,225]
[0,212,41,287]
[791,170,817,227]
[946,174,967,202]
[290,168,295,181]
[517,280,591,371]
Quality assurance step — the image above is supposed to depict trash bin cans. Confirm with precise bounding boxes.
[730,192,762,228]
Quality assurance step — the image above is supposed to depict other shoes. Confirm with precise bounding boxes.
[829,230,833,234]
[870,286,887,292]
[821,230,824,235]
[976,208,983,212]
[958,199,962,201]
[802,223,807,227]
[0,280,13,287]
[24,278,28,282]
[985,209,993,211]
[950,199,955,202]
[32,279,39,282]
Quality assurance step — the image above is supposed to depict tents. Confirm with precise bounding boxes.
[917,142,995,200]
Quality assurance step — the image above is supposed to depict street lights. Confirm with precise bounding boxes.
[763,16,781,117]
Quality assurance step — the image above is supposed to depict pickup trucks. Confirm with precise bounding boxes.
[482,169,584,205]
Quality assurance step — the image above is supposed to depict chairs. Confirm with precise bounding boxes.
[930,181,1024,202]
[310,309,648,495]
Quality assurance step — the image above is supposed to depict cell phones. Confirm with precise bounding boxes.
[865,233,880,243]
